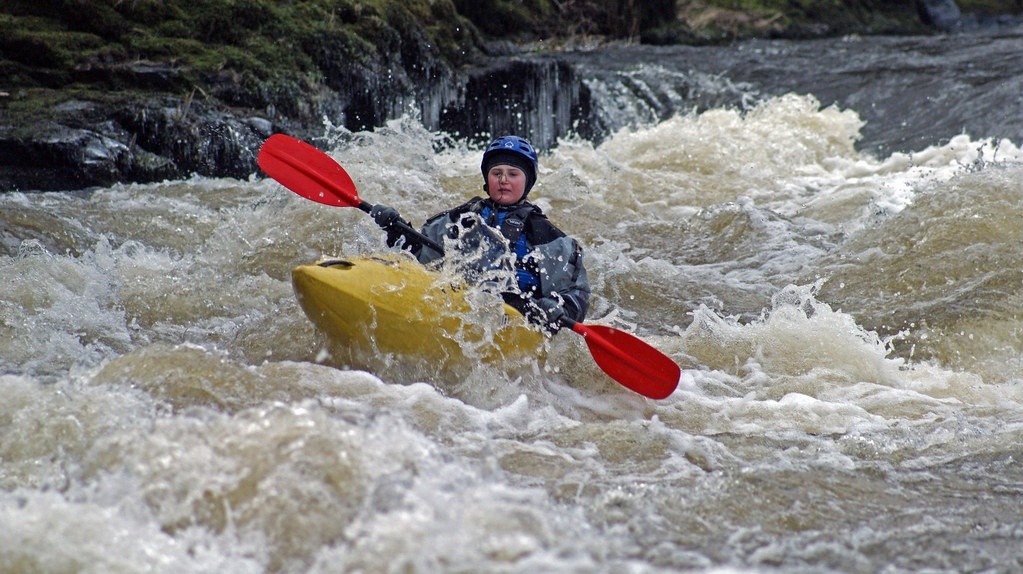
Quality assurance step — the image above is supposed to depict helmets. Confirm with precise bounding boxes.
[481,134,538,199]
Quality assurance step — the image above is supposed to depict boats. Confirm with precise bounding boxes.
[291,255,547,377]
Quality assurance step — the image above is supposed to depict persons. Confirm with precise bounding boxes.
[371,136,590,332]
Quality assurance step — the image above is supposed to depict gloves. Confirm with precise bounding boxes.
[537,297,568,328]
[371,206,405,232]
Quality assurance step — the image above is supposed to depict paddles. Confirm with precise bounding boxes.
[259,132,681,401]
